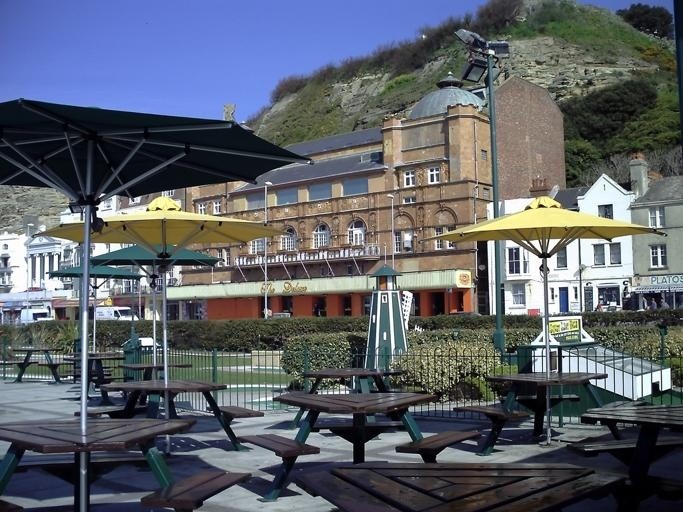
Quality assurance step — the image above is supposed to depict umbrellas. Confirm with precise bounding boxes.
[91,243,224,383]
[45,262,146,355]
[31,197,293,456]
[1,93,317,511]
[418,195,670,447]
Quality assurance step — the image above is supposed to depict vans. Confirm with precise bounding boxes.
[21,309,55,326]
[96,307,139,321]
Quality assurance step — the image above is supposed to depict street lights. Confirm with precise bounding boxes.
[385,193,395,270]
[25,222,34,308]
[263,181,272,320]
[453,28,509,348]
